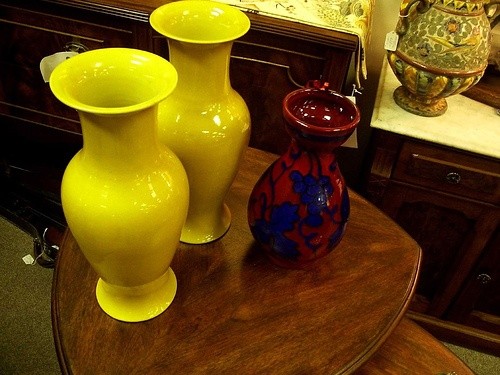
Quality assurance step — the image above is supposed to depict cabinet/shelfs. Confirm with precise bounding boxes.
[0,0,372,268]
[369,128,500,360]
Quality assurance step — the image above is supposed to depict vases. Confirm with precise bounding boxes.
[385,1,500,117]
[149,0,251,245]
[248,79,362,270]
[49,47,191,323]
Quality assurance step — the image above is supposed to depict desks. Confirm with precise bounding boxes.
[52,147,479,375]
